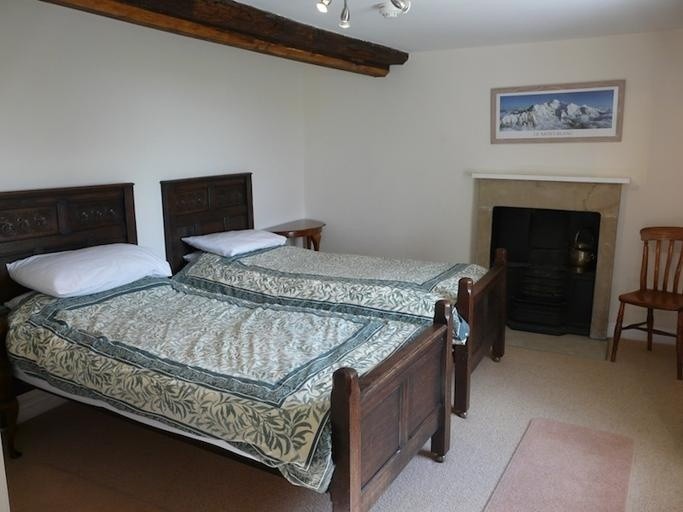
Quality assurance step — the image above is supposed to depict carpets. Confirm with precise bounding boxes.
[481,413,638,512]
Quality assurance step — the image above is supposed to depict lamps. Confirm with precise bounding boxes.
[309,0,410,29]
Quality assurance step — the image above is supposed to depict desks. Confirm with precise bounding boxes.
[261,218,328,252]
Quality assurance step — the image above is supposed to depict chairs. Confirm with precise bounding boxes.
[601,225,682,383]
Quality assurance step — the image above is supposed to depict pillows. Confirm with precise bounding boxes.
[179,224,292,259]
[1,237,175,299]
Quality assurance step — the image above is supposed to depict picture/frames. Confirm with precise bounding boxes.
[489,76,627,148]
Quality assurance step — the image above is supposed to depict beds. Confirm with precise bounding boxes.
[149,173,511,419]
[1,179,454,512]
